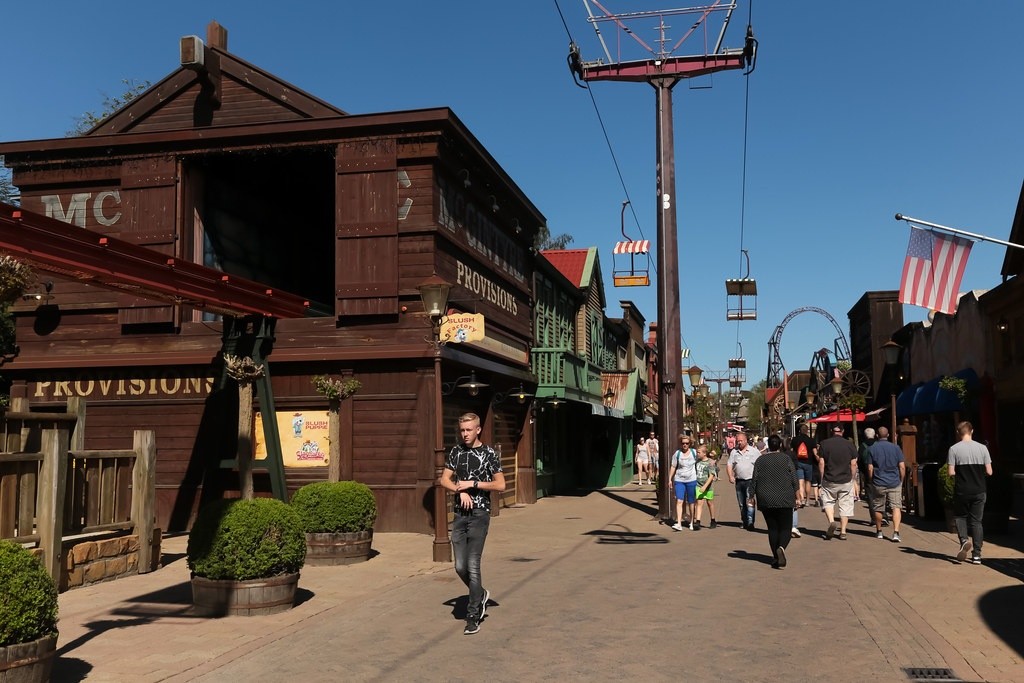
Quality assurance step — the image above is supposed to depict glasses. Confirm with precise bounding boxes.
[682,443,689,445]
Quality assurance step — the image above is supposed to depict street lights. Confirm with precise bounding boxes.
[786,399,797,438]
[758,407,787,438]
[829,376,845,423]
[698,382,717,451]
[878,336,903,446]
[804,390,816,437]
[686,366,704,451]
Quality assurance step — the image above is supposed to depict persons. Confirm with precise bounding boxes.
[789,424,824,508]
[817,422,859,543]
[723,431,801,538]
[857,426,906,543]
[667,434,722,531]
[748,434,801,570]
[634,431,659,485]
[440,413,505,632]
[946,421,992,565]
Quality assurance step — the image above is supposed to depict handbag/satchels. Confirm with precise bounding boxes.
[798,442,809,459]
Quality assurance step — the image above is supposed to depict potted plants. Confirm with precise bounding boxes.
[938,462,958,534]
[291,480,377,566]
[843,394,867,411]
[0,539,59,683]
[186,496,308,617]
[835,359,851,373]
[936,376,966,398]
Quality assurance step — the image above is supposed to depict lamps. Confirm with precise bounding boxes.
[23,281,55,307]
[531,392,566,408]
[491,382,534,409]
[995,314,1009,337]
[441,368,489,396]
[603,388,615,407]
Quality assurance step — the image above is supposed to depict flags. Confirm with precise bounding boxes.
[898,226,975,315]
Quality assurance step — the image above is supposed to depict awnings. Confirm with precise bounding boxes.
[866,406,888,422]
[895,367,981,419]
[806,406,868,422]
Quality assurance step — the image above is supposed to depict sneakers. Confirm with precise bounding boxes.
[826,523,837,539]
[479,590,490,620]
[881,514,889,525]
[777,547,786,567]
[791,533,796,537]
[973,557,981,564]
[740,522,748,529]
[870,519,876,526]
[792,527,801,537]
[748,522,754,530]
[876,532,883,539]
[892,534,901,542]
[464,618,480,634]
[957,542,972,562]
[772,562,778,569]
[839,534,847,539]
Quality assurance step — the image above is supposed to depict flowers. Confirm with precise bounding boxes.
[313,375,363,407]
[223,353,265,383]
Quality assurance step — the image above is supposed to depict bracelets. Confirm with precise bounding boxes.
[474,480,477,489]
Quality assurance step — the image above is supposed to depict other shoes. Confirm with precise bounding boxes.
[711,522,716,528]
[694,522,700,530]
[638,481,642,485]
[801,498,823,508]
[854,495,868,501]
[689,524,693,530]
[647,479,651,484]
[672,524,682,531]
